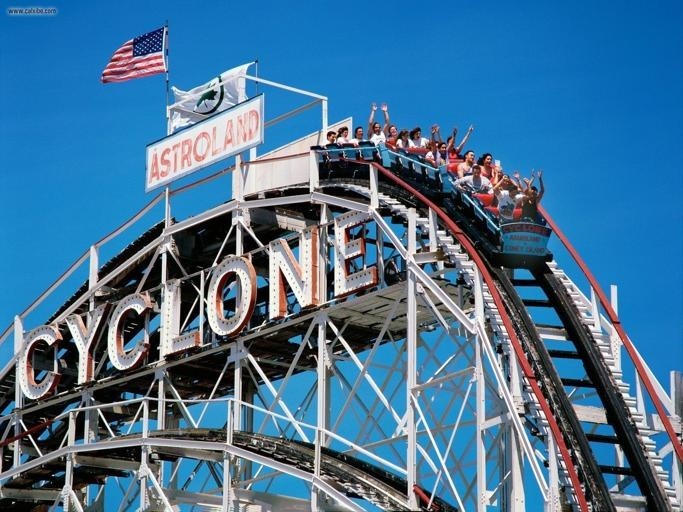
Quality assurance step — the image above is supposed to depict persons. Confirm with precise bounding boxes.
[326,99,544,226]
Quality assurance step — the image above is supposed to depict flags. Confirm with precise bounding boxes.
[97,19,169,84]
[167,57,257,133]
